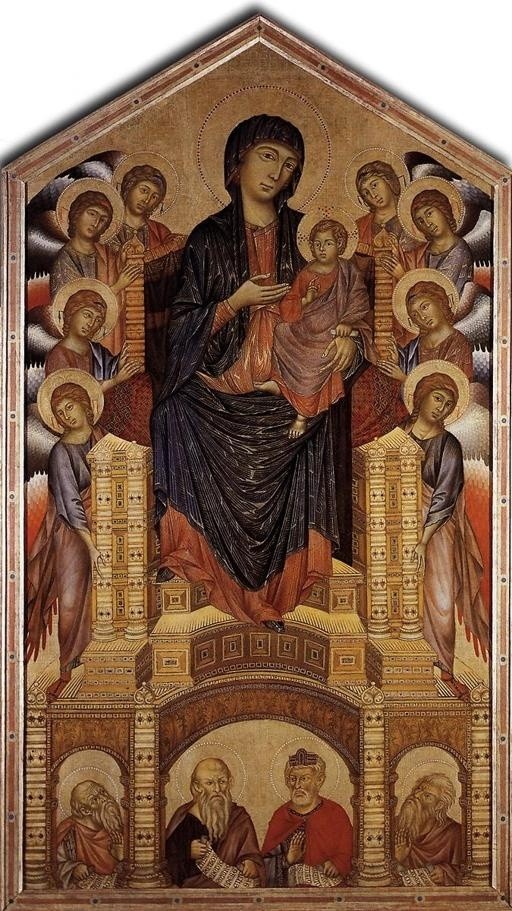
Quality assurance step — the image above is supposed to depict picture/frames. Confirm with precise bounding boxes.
[0,6,512,909]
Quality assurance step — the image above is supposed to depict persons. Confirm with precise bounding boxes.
[388,774,466,885]
[374,189,477,298]
[36,290,144,393]
[150,112,365,636]
[376,281,475,387]
[248,218,373,441]
[108,165,185,255]
[345,161,416,255]
[45,191,141,358]
[48,781,128,890]
[37,381,115,681]
[164,756,266,888]
[260,748,360,887]
[400,372,468,683]
